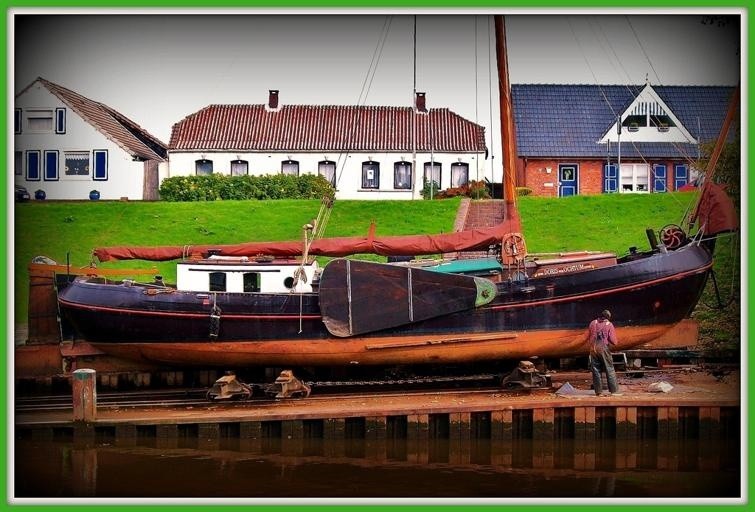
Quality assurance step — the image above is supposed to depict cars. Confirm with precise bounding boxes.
[678,177,703,192]
[15,184,31,201]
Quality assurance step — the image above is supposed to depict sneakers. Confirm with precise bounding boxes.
[611,392,624,396]
[596,392,611,397]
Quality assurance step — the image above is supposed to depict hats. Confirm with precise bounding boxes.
[602,309,612,320]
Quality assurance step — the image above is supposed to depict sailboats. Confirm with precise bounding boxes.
[52,17,717,392]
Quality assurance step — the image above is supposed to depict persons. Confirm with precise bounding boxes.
[584,310,624,395]
